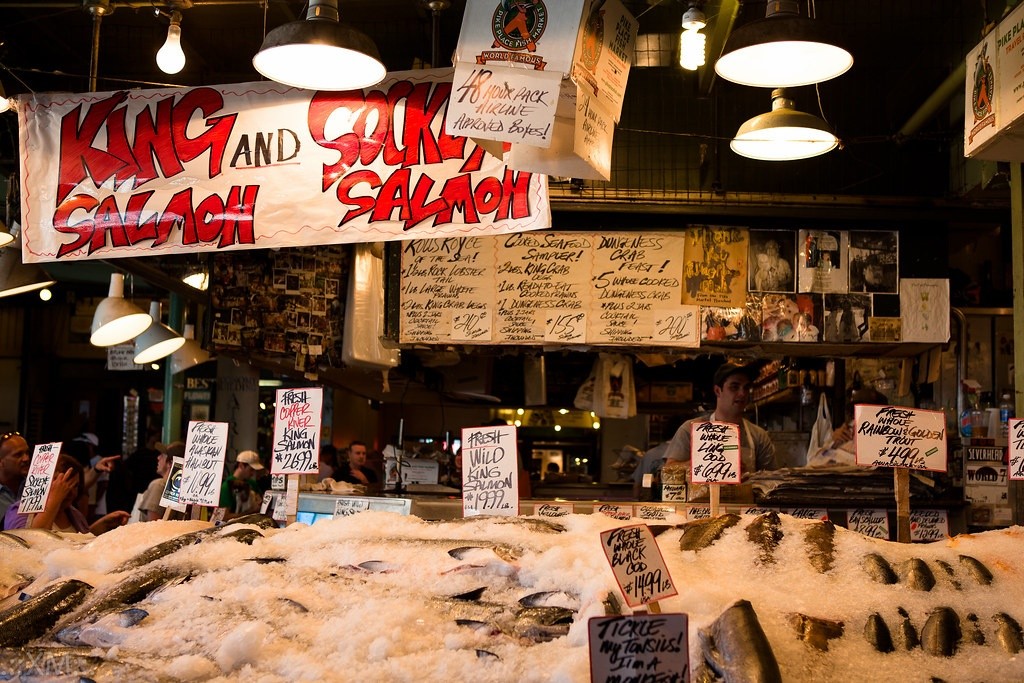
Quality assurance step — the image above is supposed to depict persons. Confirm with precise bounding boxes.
[203,243,349,379]
[662,364,779,479]
[706,238,898,341]
[0,431,467,538]
[631,416,683,499]
[812,388,891,468]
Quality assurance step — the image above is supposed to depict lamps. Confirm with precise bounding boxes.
[252,0,387,91]
[89,270,153,347]
[0,207,57,297]
[730,88,840,162]
[133,283,186,364]
[170,300,207,375]
[713,0,855,88]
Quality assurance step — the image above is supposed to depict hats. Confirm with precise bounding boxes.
[155,440,185,459]
[236,450,264,471]
[712,361,762,388]
[84,432,98,448]
[854,388,886,407]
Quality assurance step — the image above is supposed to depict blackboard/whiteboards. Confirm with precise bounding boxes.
[399,228,701,348]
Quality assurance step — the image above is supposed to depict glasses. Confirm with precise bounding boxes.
[1,430,21,448]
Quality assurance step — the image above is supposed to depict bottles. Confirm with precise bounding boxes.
[998,393,1015,439]
[971,410,983,440]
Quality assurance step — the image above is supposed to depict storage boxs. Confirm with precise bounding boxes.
[385,457,439,487]
